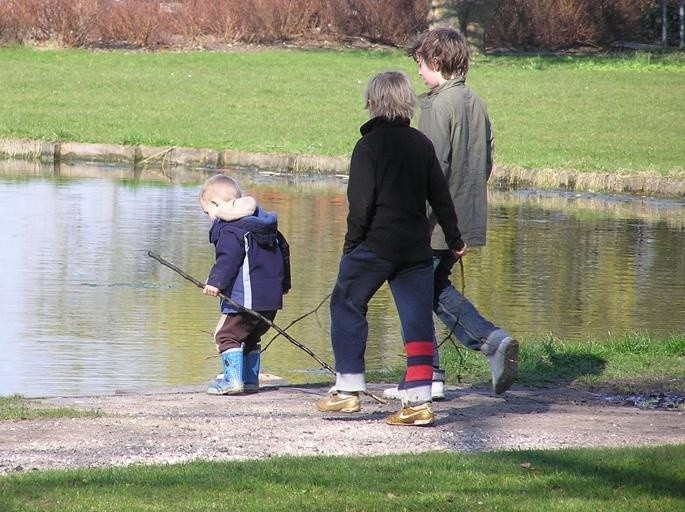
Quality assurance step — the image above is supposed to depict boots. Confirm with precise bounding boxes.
[208,347,244,395]
[244,352,261,392]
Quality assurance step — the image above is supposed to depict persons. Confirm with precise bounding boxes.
[317,71,469,426]
[199,174,292,396]
[383,26,520,402]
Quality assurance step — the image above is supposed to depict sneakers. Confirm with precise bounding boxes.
[488,337,519,393]
[385,406,434,426]
[381,381,445,402]
[316,395,360,413]
